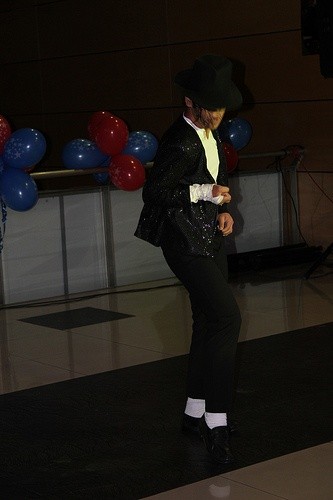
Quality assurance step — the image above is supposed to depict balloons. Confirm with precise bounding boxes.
[62,138,108,170]
[75,150,108,185]
[223,118,252,151]
[222,142,239,175]
[126,132,158,162]
[0,115,12,155]
[108,154,145,191]
[2,128,46,169]
[88,111,112,142]
[95,116,129,158]
[0,169,38,211]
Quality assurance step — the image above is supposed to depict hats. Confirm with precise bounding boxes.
[183,53,243,110]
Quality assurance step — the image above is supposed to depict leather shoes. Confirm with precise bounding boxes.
[180,412,240,438]
[197,414,235,465]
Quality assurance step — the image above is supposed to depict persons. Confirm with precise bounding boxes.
[134,54,244,466]
